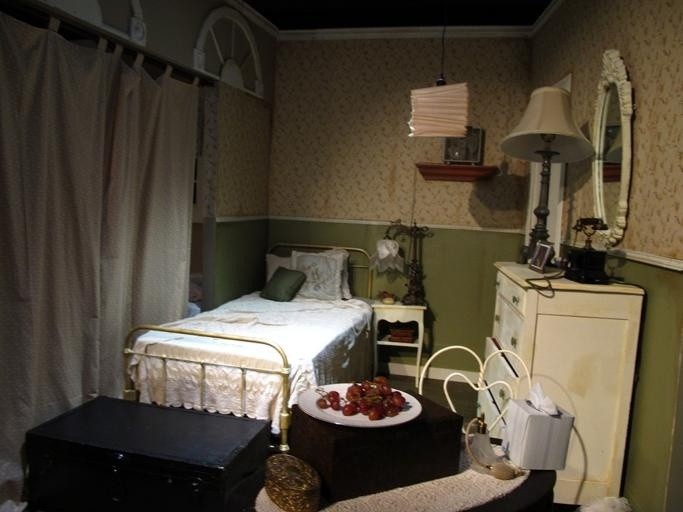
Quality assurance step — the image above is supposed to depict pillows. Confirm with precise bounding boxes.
[259,247,354,301]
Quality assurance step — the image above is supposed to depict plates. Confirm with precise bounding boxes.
[297,383,424,428]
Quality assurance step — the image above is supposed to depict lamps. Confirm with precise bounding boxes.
[606,127,623,163]
[500,88,595,264]
[369,218,431,305]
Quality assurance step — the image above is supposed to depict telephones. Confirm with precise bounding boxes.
[560,217,611,283]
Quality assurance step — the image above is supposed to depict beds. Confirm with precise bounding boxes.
[123,241,373,455]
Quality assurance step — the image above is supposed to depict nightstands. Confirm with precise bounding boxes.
[372,301,428,387]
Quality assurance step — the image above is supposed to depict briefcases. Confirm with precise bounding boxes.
[21,394,273,511]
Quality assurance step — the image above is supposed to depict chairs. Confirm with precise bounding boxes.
[418,346,532,439]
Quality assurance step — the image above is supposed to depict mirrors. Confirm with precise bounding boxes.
[593,46,633,246]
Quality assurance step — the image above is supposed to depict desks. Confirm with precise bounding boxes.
[253,434,557,512]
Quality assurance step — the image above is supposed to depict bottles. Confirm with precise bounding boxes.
[472,423,490,473]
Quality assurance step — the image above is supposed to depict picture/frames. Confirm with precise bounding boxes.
[528,241,552,273]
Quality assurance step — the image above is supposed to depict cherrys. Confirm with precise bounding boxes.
[314,377,405,420]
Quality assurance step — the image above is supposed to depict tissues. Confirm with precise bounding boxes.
[501,381,575,471]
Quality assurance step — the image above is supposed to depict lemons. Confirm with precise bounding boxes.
[382,298,394,304]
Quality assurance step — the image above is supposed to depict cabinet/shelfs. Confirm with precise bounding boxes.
[475,261,646,512]
